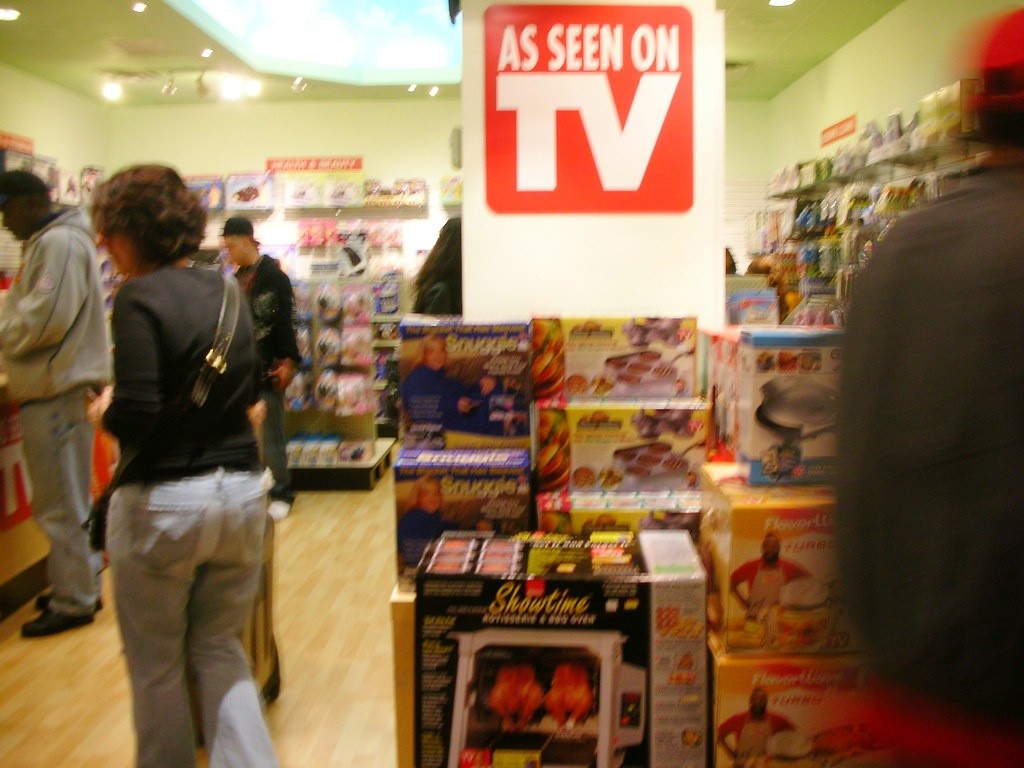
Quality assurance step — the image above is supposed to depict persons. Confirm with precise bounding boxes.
[729,533,814,623]
[88,166,275,768]
[746,260,789,320]
[718,688,797,768]
[222,216,302,521]
[412,217,462,315]
[402,334,503,437]
[0,169,110,637]
[829,10,1024,731]
[397,477,490,570]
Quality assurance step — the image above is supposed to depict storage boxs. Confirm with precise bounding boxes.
[703,463,853,658]
[639,529,709,768]
[394,450,532,592]
[741,325,846,484]
[705,631,1006,768]
[536,492,704,536]
[401,313,534,451]
[534,313,698,400]
[536,399,712,491]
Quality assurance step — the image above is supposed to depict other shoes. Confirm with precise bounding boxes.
[35,590,103,613]
[22,607,94,638]
[266,499,291,520]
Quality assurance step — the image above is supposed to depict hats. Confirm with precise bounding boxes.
[968,8,1024,108]
[219,216,261,248]
[0,169,49,211]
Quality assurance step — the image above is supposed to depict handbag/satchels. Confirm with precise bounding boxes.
[81,478,118,553]
